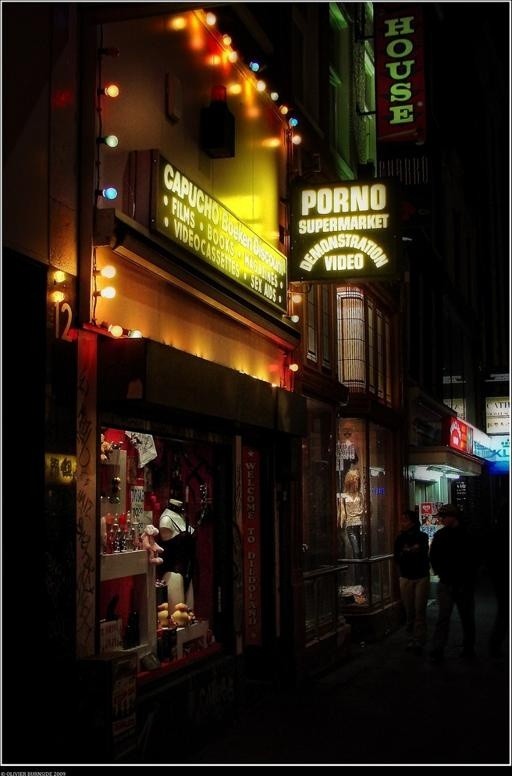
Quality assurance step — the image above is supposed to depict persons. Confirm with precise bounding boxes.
[337,470,370,588]
[393,511,430,647]
[423,514,434,526]
[159,498,196,618]
[432,504,482,649]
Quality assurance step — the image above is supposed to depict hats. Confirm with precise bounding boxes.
[433,503,461,517]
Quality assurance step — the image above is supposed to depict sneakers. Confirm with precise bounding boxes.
[406,624,428,640]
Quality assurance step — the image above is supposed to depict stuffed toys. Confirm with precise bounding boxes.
[140,525,165,565]
